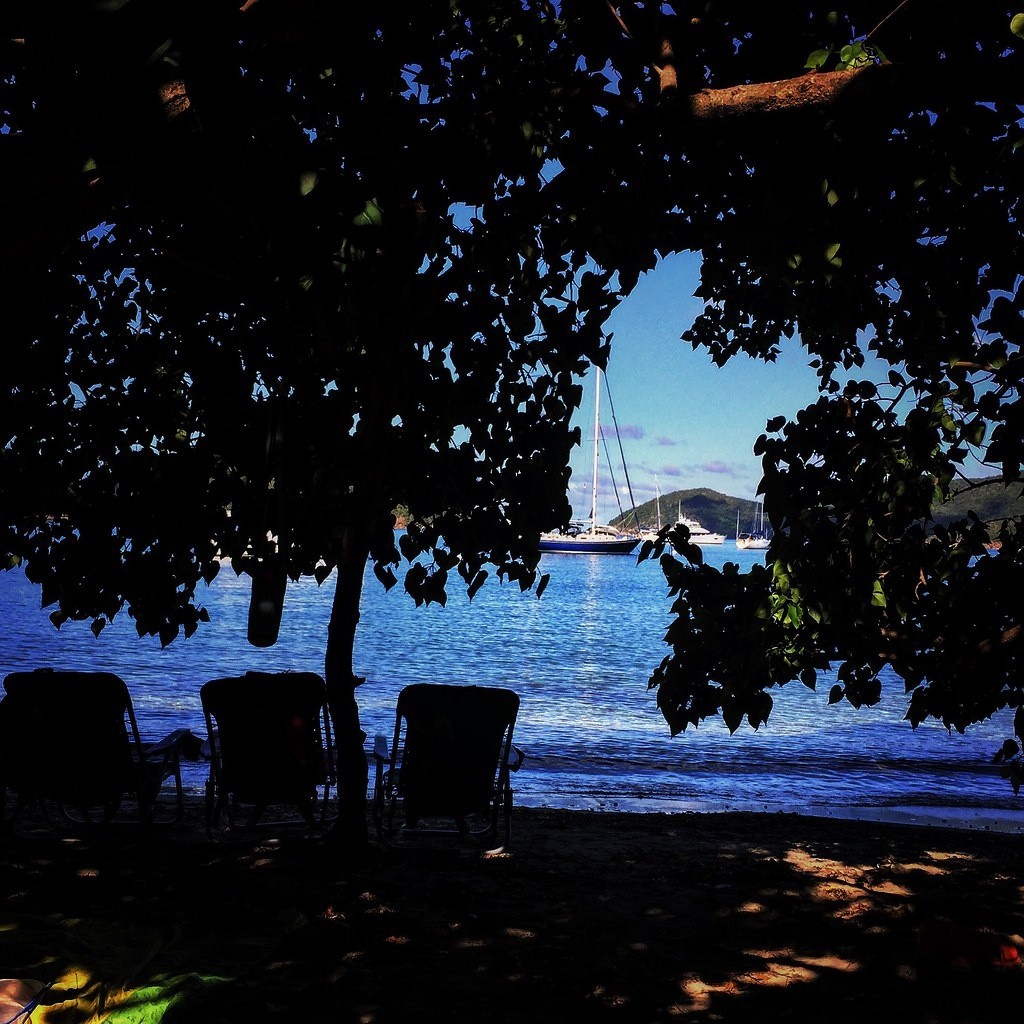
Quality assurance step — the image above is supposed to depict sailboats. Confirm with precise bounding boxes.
[736,482,772,550]
[537,350,643,556]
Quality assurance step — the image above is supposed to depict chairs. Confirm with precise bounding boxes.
[368,681,524,864]
[197,671,338,848]
[0,670,184,847]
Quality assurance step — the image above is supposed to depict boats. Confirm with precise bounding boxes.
[639,472,728,545]
